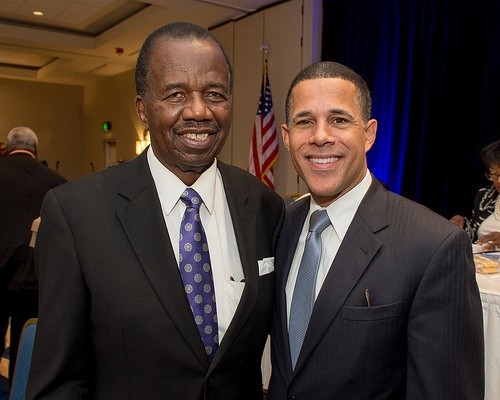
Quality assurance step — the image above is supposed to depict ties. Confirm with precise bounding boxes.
[289,206,330,370]
[179,188,219,363]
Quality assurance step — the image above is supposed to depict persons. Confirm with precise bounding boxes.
[449,141,500,251]
[0,142,8,157]
[0,126,68,400]
[24,22,286,400]
[267,62,485,400]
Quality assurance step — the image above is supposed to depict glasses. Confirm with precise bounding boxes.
[485,172,500,180]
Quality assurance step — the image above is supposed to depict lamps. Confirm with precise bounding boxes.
[136,140,151,155]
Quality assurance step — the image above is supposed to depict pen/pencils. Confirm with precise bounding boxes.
[365,287,371,307]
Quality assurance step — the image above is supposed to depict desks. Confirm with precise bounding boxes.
[475,251,500,400]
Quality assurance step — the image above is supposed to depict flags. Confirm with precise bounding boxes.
[245,66,279,191]
